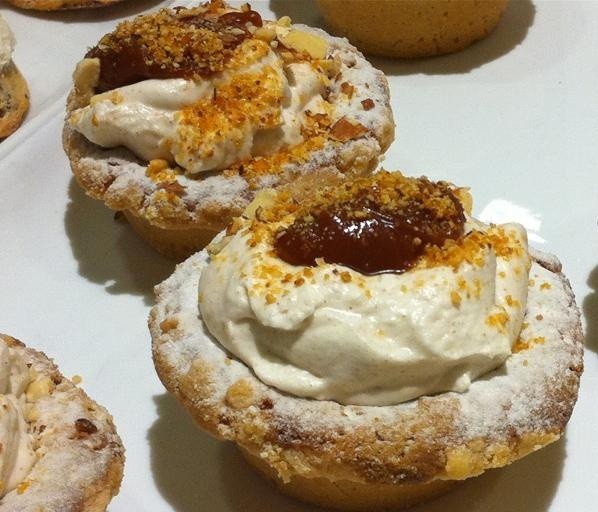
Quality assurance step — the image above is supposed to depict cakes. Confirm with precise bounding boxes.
[0,12,30,140]
[148,166,584,510]
[8,0,122,11]
[311,0,512,59]
[62,0,396,260]
[0,333,126,512]
